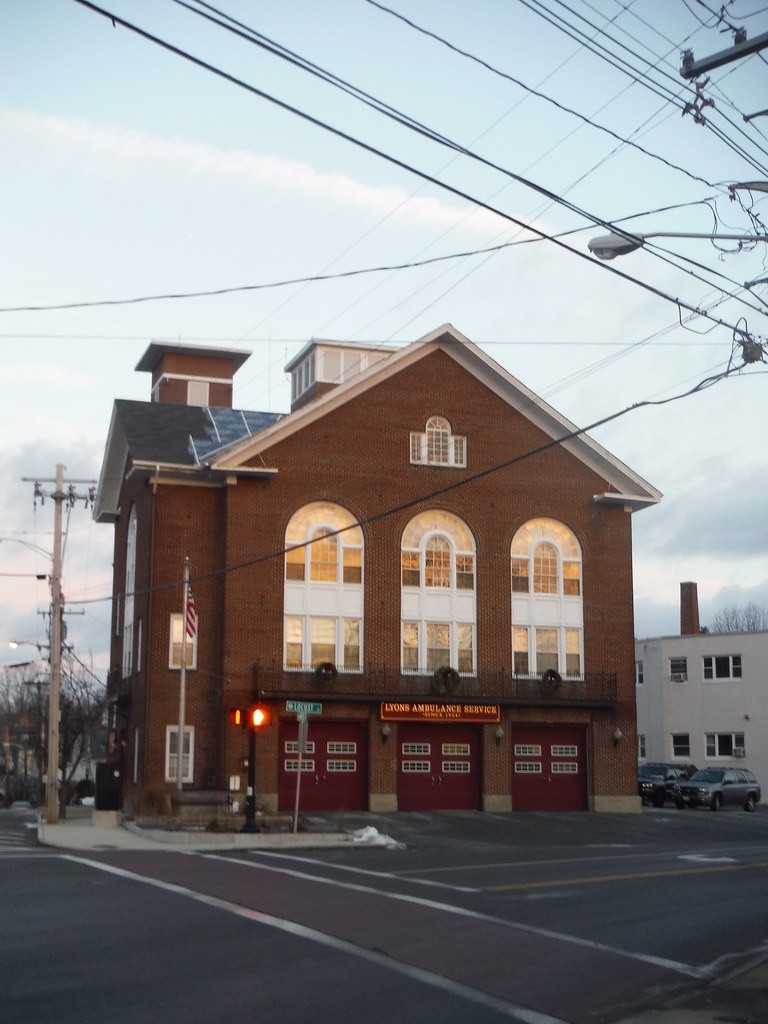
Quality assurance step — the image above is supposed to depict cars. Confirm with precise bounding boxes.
[10,799,32,811]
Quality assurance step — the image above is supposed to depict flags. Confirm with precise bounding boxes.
[187,572,196,636]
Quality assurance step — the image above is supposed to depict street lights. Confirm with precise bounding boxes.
[8,640,63,825]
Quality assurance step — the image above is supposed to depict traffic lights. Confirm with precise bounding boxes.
[231,708,244,726]
[250,708,266,728]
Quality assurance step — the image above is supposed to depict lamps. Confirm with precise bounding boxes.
[380,722,392,745]
[613,726,623,748]
[493,724,504,747]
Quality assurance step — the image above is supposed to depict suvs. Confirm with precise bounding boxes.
[672,766,761,812]
[638,762,700,809]
